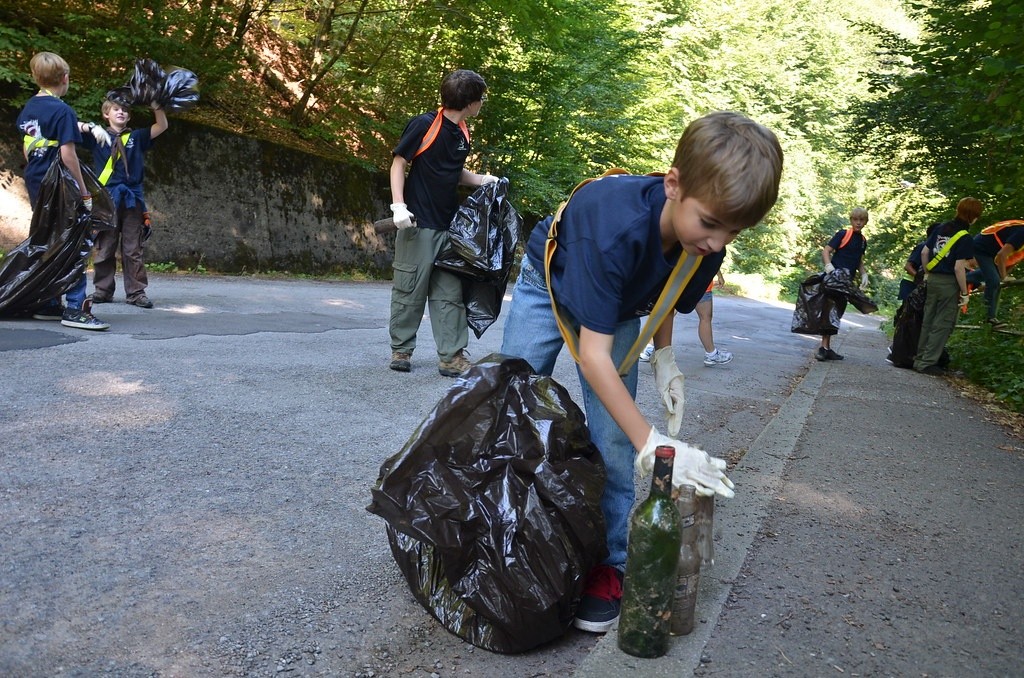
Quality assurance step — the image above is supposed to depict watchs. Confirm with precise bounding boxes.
[89,122,95,133]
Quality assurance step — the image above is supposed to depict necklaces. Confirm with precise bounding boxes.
[38,89,58,97]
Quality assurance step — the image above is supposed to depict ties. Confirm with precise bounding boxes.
[105,126,132,182]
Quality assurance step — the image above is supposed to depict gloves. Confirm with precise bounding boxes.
[480,175,499,186]
[635,425,735,498]
[860,274,869,288]
[91,124,112,149]
[922,274,928,281]
[390,203,417,231]
[83,198,92,212]
[825,263,835,275]
[650,346,684,439]
[957,292,970,307]
[1002,276,1016,282]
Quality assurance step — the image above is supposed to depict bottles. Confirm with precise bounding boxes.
[668,482,700,635]
[692,484,717,569]
[374,216,417,234]
[618,445,677,658]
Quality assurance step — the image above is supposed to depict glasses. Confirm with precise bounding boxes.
[479,93,488,102]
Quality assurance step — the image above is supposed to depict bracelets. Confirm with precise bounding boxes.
[81,123,86,133]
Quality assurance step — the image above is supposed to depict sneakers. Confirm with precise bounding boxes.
[639,345,655,362]
[572,566,624,633]
[88,292,113,303]
[60,297,111,329]
[439,349,473,376]
[983,317,1008,330]
[33,297,65,321]
[826,349,844,360]
[704,351,733,367]
[127,296,153,308]
[815,347,827,361]
[918,365,944,377]
[390,352,410,372]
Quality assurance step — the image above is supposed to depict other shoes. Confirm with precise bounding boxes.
[887,343,894,354]
[885,353,893,364]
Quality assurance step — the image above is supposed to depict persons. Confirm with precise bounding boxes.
[501,109,785,633]
[387,69,509,378]
[76,86,169,308]
[17,52,111,330]
[639,268,734,366]
[815,207,869,362]
[885,196,1024,376]
